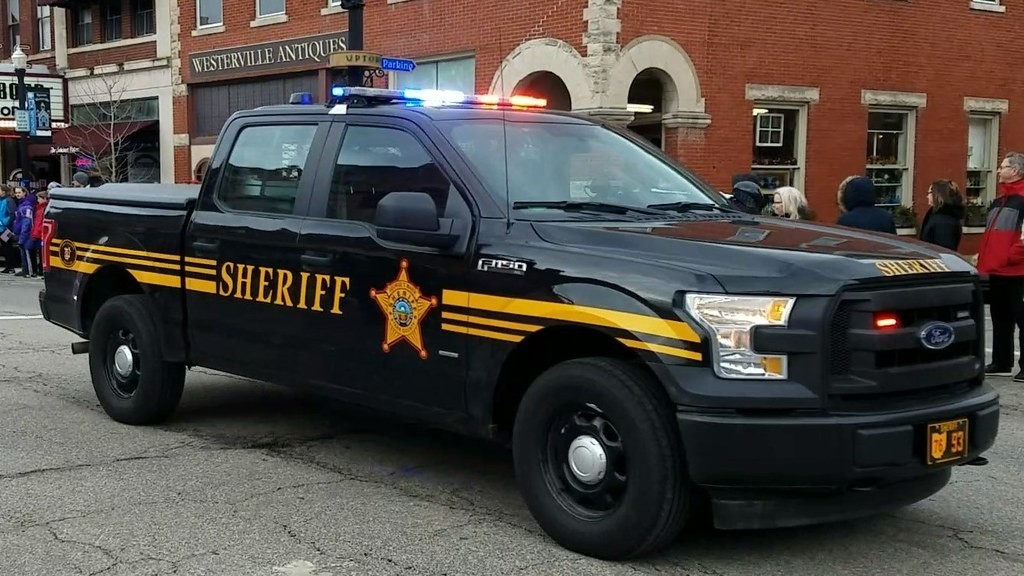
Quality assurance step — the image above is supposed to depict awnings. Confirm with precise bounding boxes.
[24,120,155,157]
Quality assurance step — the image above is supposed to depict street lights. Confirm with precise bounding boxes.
[12,45,29,191]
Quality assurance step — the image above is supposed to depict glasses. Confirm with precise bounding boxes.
[999,166,1013,170]
[927,191,933,194]
[772,200,783,205]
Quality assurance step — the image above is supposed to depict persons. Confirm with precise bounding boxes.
[975,153,1024,381]
[773,186,816,221]
[837,175,897,236]
[917,180,965,252]
[0,171,91,282]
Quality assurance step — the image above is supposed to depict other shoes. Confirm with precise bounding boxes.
[983,363,1015,378]
[13,272,37,278]
[1012,370,1024,382]
[9,270,15,274]
[0,270,10,274]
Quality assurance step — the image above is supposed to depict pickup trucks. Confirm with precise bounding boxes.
[37,85,1004,563]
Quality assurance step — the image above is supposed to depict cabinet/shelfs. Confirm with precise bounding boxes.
[966,168,992,189]
[865,164,908,206]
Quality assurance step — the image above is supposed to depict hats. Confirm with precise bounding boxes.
[73,172,89,185]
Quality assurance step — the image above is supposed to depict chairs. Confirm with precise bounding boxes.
[344,154,379,223]
